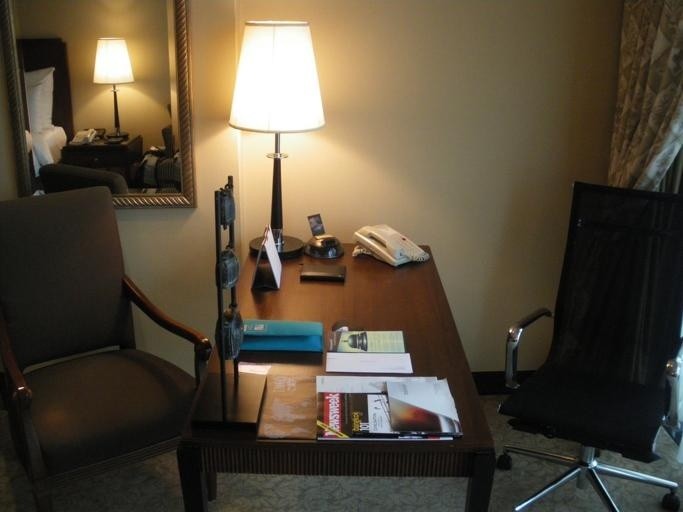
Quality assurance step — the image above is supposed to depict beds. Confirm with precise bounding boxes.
[16,38,74,192]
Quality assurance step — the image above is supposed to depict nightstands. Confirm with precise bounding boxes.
[62,134,147,189]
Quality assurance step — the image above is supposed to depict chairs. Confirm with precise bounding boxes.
[0,186,217,512]
[39,163,129,195]
[496,181,683,512]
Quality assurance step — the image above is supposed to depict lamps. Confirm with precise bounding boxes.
[227,20,326,260]
[92,37,136,140]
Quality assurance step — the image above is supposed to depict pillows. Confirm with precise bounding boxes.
[23,66,56,134]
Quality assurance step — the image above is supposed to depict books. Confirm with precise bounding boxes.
[313,329,465,442]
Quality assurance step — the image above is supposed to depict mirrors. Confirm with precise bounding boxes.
[0,0,198,208]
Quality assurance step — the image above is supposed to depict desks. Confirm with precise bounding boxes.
[176,243,496,512]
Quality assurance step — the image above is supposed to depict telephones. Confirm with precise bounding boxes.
[354,224,427,267]
